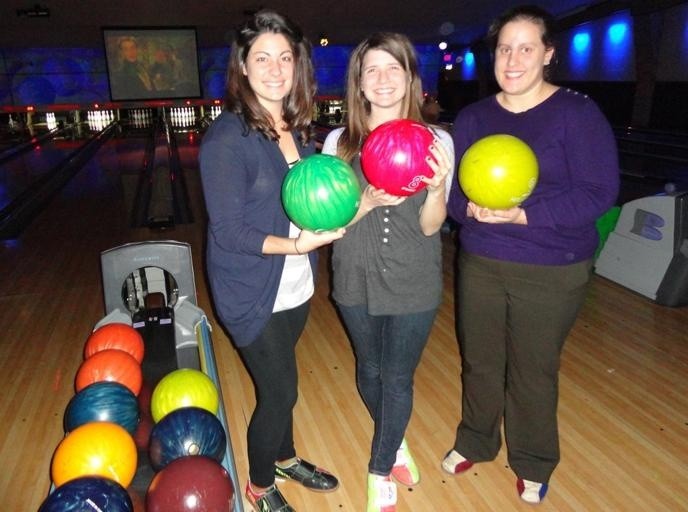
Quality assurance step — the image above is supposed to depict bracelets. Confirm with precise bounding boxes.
[294,238,301,256]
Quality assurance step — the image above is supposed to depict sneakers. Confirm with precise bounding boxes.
[516,478,548,504]
[442,449,474,475]
[389,438,420,486]
[366,473,398,512]
[274,457,339,493]
[245,477,296,512]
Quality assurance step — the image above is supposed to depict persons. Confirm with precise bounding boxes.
[115,37,155,94]
[199,10,348,512]
[436,2,620,506]
[317,29,457,511]
